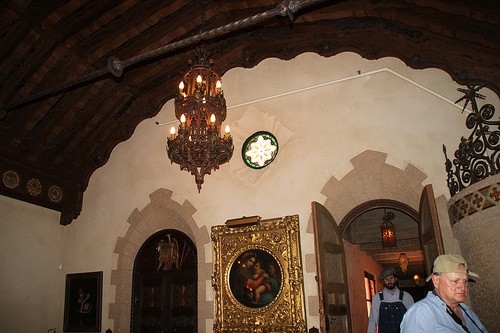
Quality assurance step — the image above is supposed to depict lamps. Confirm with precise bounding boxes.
[398,253,410,273]
[226,216,261,228]
[379,208,397,250]
[165,0,234,194]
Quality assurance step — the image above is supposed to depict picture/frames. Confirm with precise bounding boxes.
[210,215,308,333]
[63,271,103,333]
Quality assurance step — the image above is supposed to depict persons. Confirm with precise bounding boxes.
[367,267,414,333]
[400,254,488,333]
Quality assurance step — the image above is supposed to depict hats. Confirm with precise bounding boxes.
[426,254,479,282]
[379,267,396,280]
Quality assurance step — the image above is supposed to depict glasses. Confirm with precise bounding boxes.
[439,275,468,287]
